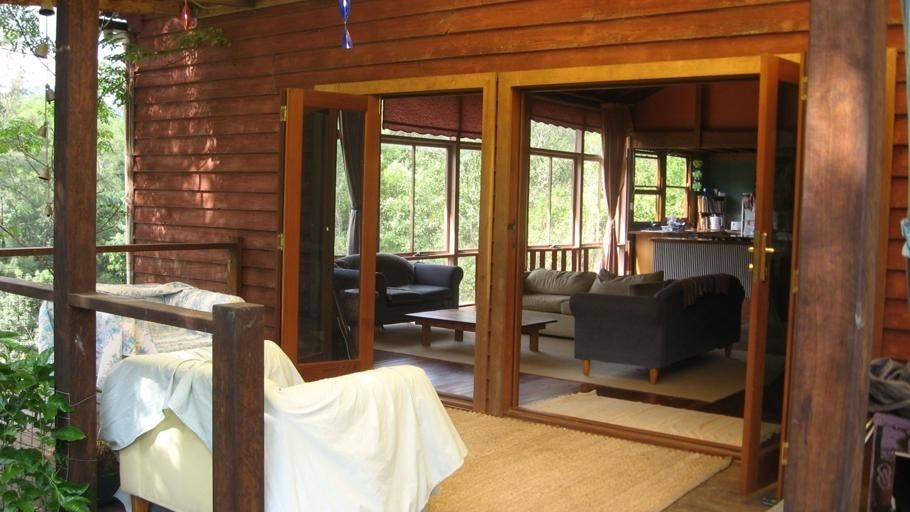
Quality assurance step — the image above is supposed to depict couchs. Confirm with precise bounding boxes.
[321,255,466,332]
[97,342,463,512]
[523,266,668,342]
[566,273,744,383]
[37,277,252,440]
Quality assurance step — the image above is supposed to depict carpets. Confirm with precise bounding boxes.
[362,323,786,404]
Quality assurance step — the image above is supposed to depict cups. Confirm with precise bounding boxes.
[710,216,724,230]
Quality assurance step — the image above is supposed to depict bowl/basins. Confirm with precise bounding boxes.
[661,226,673,232]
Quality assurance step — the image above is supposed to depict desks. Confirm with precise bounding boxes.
[405,307,561,352]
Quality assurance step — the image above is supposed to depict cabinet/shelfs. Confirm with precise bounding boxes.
[629,229,791,299]
